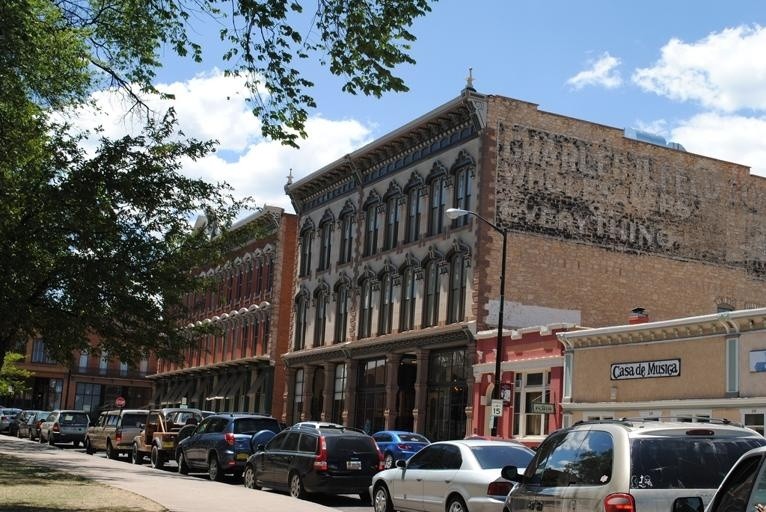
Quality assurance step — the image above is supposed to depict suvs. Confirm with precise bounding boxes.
[131,407,206,470]
[0,407,52,441]
[242,427,384,502]
[83,408,152,459]
[174,413,284,482]
[501,418,766,512]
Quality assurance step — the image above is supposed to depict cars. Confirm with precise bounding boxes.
[291,422,346,430]
[39,409,90,447]
[368,437,543,512]
[369,430,432,470]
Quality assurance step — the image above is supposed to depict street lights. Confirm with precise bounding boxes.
[445,207,508,439]
